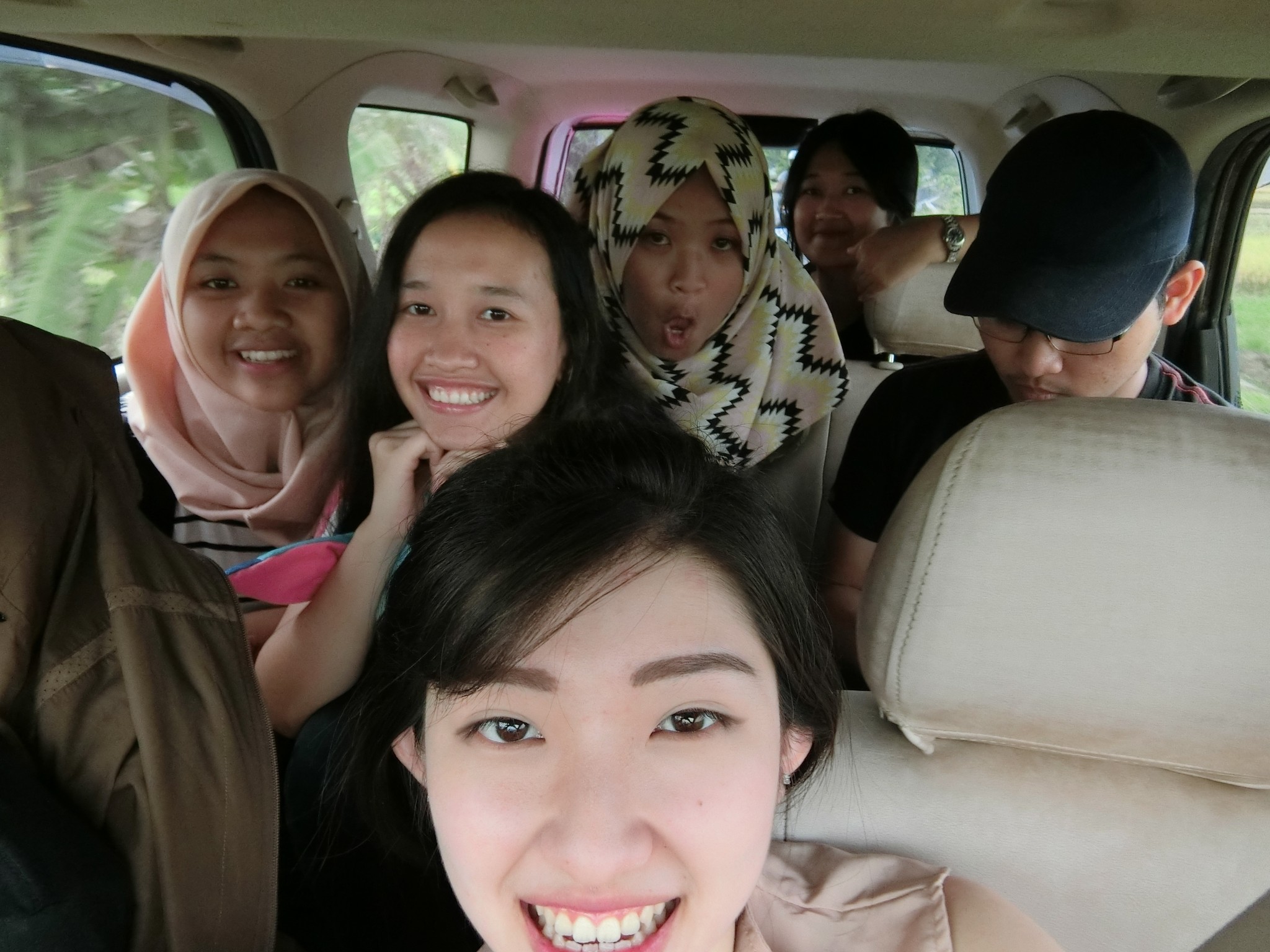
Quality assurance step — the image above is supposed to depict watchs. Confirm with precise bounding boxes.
[935,214,966,263]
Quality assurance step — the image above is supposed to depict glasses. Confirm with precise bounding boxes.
[966,310,1125,356]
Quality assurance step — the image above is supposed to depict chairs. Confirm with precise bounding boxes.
[771,398,1269,950]
[774,260,979,589]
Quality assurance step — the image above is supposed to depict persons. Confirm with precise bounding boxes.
[332,423,1077,952]
[255,170,621,737]
[566,94,847,474]
[118,169,372,650]
[821,107,1241,689]
[778,109,980,359]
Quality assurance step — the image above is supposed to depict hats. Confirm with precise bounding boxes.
[941,106,1192,340]
